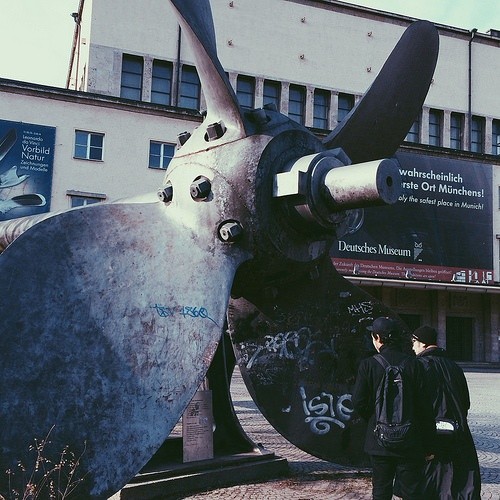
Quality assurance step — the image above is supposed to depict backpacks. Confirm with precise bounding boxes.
[373,353,416,443]
[431,381,471,464]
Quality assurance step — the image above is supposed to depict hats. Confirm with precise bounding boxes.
[366,316,399,332]
[411,325,437,345]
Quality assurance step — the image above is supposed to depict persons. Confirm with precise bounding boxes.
[404,322,485,500]
[351,315,426,500]
